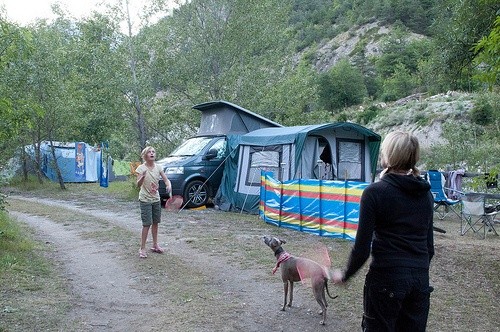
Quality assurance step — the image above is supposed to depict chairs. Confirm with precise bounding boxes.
[460,192,500,239]
[425,170,461,220]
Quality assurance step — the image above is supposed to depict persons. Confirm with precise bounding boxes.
[134,146,172,258]
[331,131,435,332]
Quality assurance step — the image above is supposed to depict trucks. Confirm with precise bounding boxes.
[155,100,285,207]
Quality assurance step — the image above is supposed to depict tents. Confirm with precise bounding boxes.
[213,122,381,215]
[20,140,113,188]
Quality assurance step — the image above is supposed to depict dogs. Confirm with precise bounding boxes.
[263,235,339,326]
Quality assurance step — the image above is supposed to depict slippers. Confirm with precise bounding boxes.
[139,247,148,258]
[150,246,163,253]
